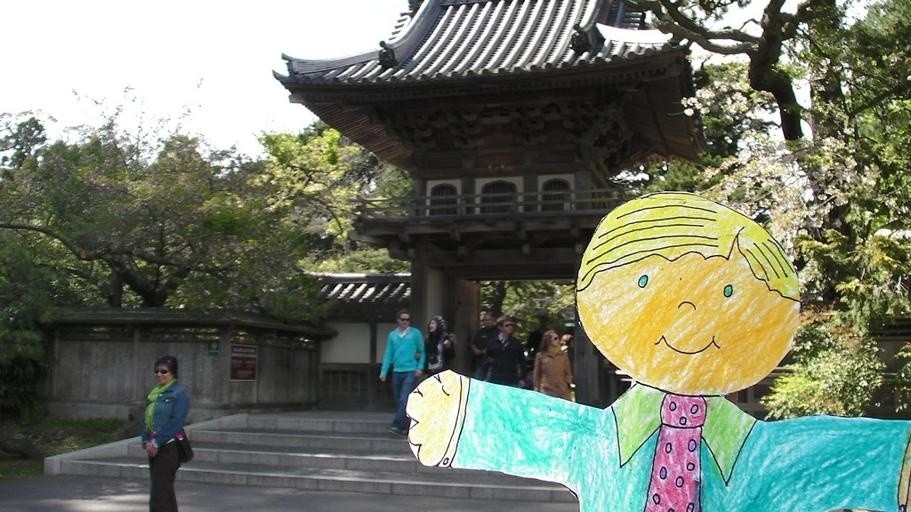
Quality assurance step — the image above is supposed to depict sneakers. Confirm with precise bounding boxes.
[388,426,410,439]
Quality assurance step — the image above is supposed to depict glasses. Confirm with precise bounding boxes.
[153,369,172,374]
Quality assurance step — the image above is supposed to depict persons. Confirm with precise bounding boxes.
[421,315,459,378]
[532,329,577,403]
[138,353,191,512]
[376,308,427,435]
[559,333,577,379]
[469,312,500,380]
[482,315,528,389]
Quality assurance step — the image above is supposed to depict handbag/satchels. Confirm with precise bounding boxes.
[175,430,194,463]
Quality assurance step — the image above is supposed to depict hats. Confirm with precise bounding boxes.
[432,315,447,330]
